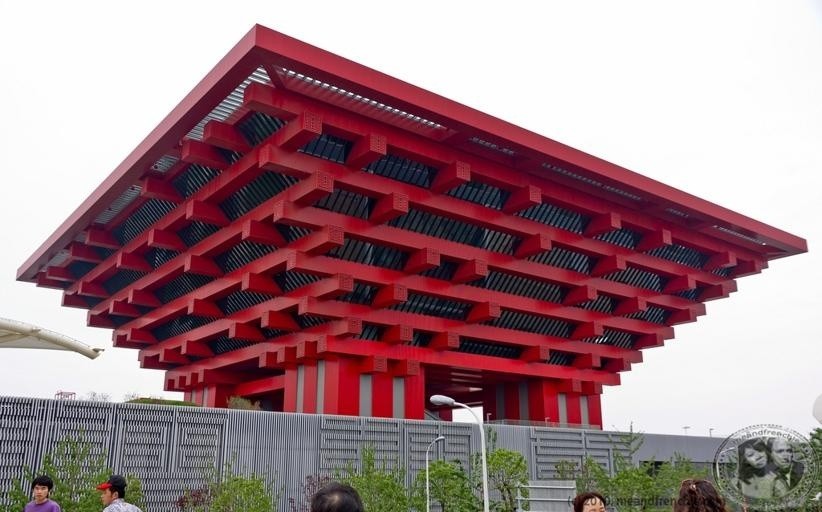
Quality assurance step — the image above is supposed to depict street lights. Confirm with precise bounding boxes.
[425,435,446,512]
[429,392,491,512]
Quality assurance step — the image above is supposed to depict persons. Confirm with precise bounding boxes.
[22,476,63,510]
[669,476,727,511]
[766,435,804,492]
[733,437,789,499]
[93,474,144,511]
[572,488,611,512]
[309,481,365,511]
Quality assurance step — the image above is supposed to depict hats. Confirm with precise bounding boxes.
[96,475,127,490]
